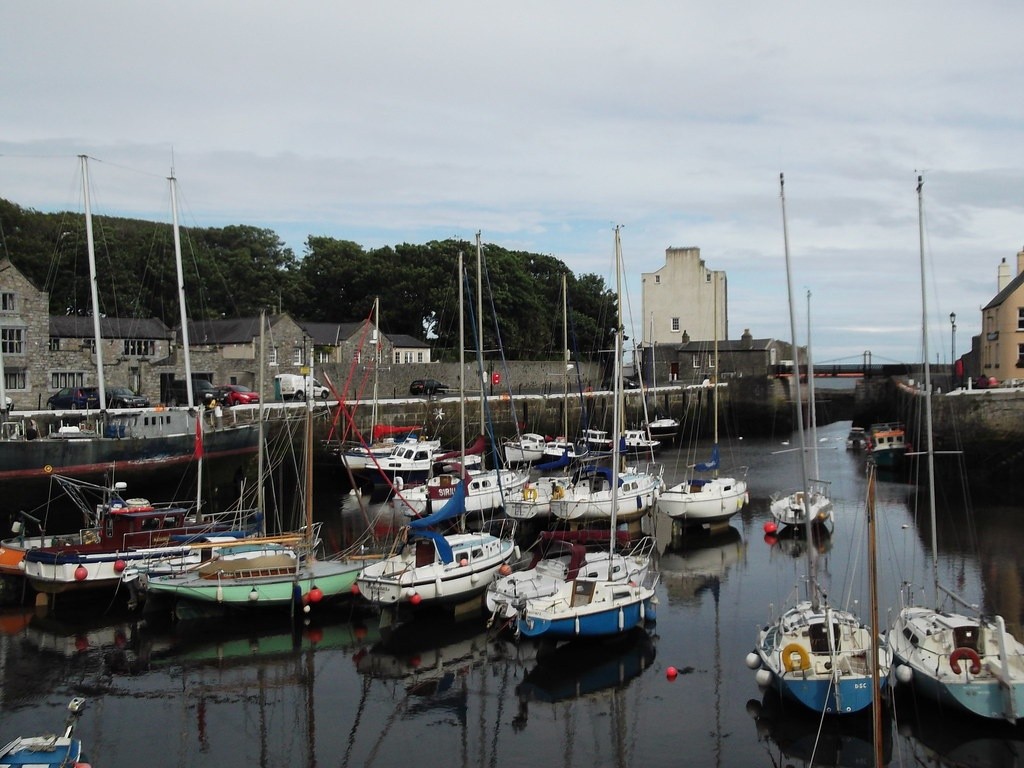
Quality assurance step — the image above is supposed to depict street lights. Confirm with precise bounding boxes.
[949,311,957,390]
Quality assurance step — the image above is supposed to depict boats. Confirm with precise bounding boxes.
[846,427,868,450]
[0,696,95,768]
[867,420,913,470]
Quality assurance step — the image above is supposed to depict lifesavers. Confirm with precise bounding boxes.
[950,647,981,674]
[111,507,153,513]
[524,487,537,501]
[419,436,425,441]
[782,643,810,671]
[553,486,564,499]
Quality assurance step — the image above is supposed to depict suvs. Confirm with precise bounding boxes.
[168,379,228,405]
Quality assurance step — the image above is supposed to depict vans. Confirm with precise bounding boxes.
[275,374,330,401]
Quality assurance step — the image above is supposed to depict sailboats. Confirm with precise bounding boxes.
[4,149,1022,723]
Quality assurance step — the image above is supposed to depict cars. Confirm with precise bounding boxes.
[410,378,450,394]
[105,385,152,408]
[46,386,101,409]
[603,375,639,389]
[216,384,259,404]
